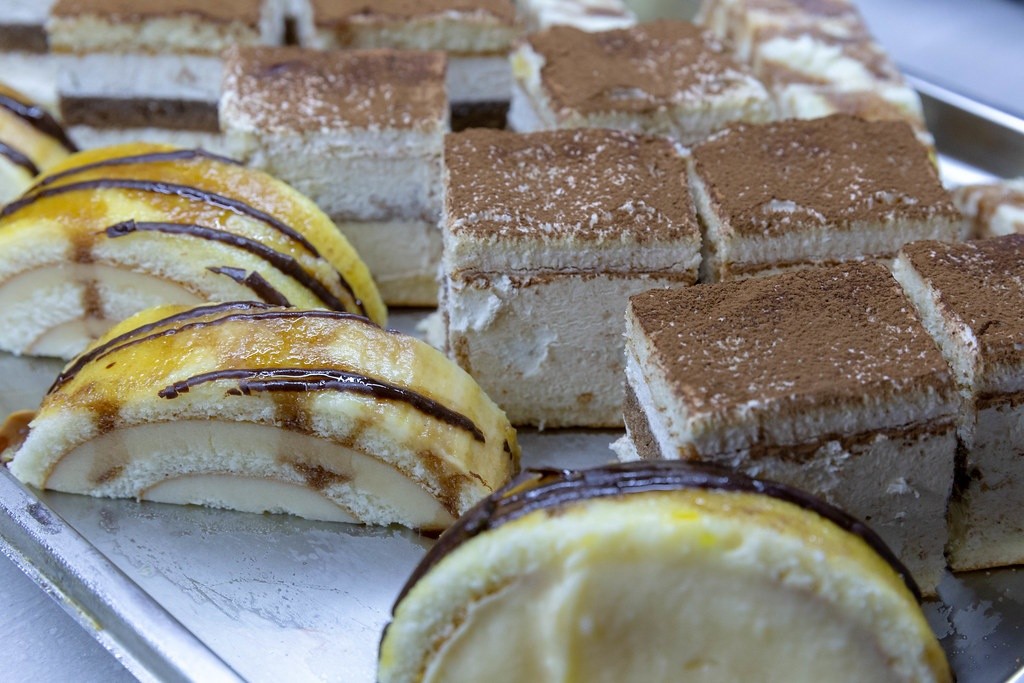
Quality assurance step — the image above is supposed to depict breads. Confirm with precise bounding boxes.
[1,0,1023,683]
